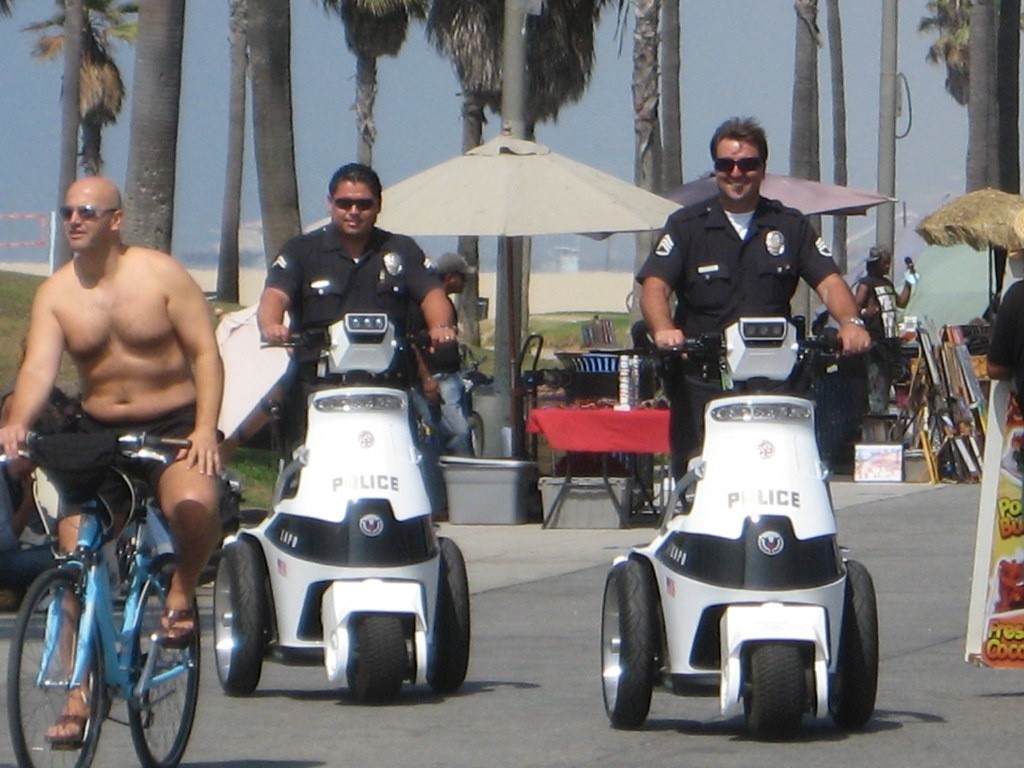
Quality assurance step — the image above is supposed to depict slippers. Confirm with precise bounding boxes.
[159,602,201,650]
[45,713,87,744]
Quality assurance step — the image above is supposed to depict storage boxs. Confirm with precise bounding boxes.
[537,472,638,527]
[440,454,539,526]
[853,441,904,482]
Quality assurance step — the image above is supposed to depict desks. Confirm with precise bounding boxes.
[527,407,673,530]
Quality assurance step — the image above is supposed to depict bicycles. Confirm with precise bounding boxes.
[0,431,246,768]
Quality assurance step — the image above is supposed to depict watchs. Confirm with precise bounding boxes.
[841,317,865,328]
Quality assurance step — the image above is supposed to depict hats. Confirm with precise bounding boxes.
[865,245,889,264]
[437,253,477,274]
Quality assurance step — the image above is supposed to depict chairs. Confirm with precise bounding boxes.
[544,349,663,531]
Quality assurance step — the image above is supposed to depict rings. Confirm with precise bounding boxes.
[445,336,450,340]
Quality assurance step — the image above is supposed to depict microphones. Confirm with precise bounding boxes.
[905,257,915,276]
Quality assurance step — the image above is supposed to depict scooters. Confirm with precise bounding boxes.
[213,312,478,704]
[592,314,880,748]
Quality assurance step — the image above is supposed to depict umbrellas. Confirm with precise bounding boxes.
[211,303,294,444]
[915,186,1024,323]
[300,121,685,460]
[575,175,899,239]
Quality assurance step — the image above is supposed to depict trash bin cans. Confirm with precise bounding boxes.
[817,353,869,475]
[555,351,649,476]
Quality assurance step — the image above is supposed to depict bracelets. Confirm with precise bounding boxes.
[428,325,458,335]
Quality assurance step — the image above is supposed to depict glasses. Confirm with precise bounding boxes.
[715,157,758,170]
[335,197,375,210]
[61,206,116,220]
[455,271,466,279]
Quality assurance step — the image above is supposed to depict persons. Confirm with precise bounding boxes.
[237,163,476,520]
[856,245,919,443]
[0,177,225,743]
[640,116,870,484]
[987,278,1024,418]
